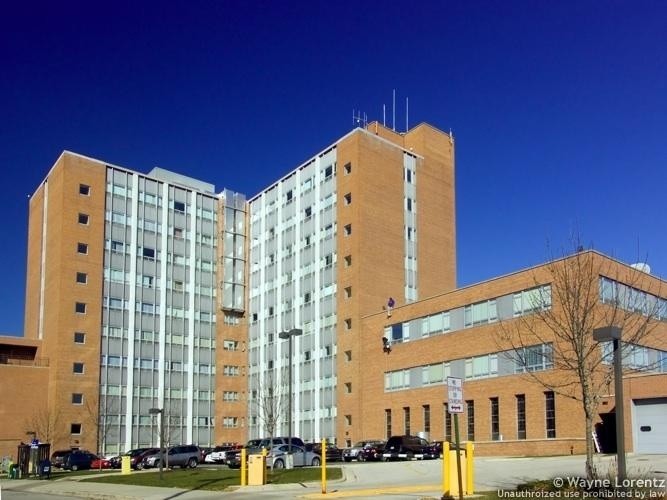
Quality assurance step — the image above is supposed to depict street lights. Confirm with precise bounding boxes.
[279,328,303,452]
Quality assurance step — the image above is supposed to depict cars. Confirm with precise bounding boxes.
[52,444,202,470]
[201,435,467,470]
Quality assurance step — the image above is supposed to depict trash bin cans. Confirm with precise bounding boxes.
[121,456,131,475]
[450,448,468,496]
[38,460,51,479]
[248,455,267,485]
[9,464,19,479]
